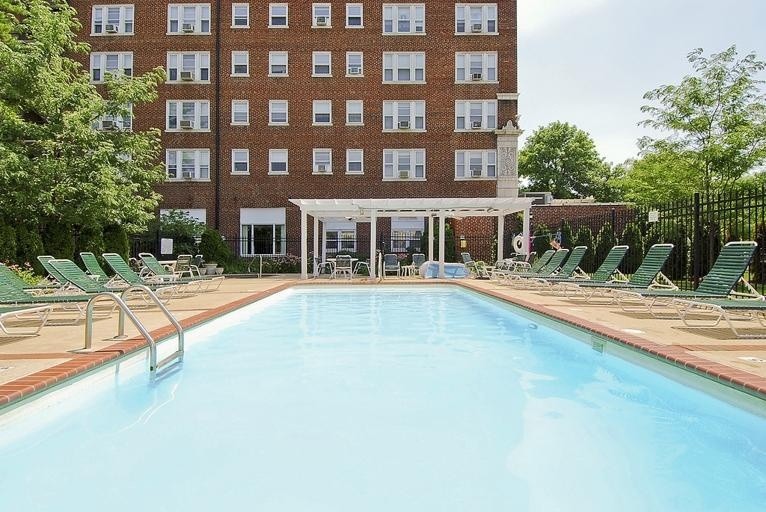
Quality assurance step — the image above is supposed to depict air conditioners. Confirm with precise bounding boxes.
[350,67,359,73]
[102,121,116,128]
[318,164,326,172]
[399,120,409,129]
[471,170,482,178]
[180,120,193,128]
[180,72,193,80]
[316,17,326,25]
[473,23,482,32]
[472,72,482,81]
[399,170,409,178]
[183,171,191,179]
[182,23,194,32]
[471,121,481,129]
[106,24,118,33]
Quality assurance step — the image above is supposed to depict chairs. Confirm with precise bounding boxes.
[314,255,370,279]
[0,252,225,335]
[383,254,425,280]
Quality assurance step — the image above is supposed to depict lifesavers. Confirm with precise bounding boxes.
[513,235,525,253]
